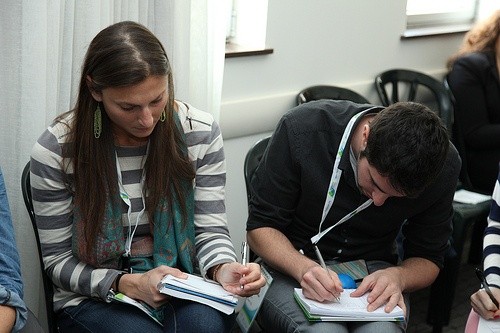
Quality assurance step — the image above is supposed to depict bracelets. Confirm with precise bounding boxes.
[213,264,220,280]
[116,272,128,293]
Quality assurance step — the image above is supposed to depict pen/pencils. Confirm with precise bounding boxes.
[475,267,499,308]
[240,240,248,289]
[314,245,339,299]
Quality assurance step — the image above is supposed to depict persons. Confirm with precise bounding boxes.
[247,100,462,333]
[447,11,500,193]
[0,167,45,333]
[464,176,500,333]
[30,21,266,333]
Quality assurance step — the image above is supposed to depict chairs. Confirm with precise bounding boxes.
[297,86,371,106]
[375,69,453,132]
[20,160,57,333]
[243,136,278,263]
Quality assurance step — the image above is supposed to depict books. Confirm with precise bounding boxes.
[453,190,491,209]
[293,288,404,322]
[157,272,238,315]
